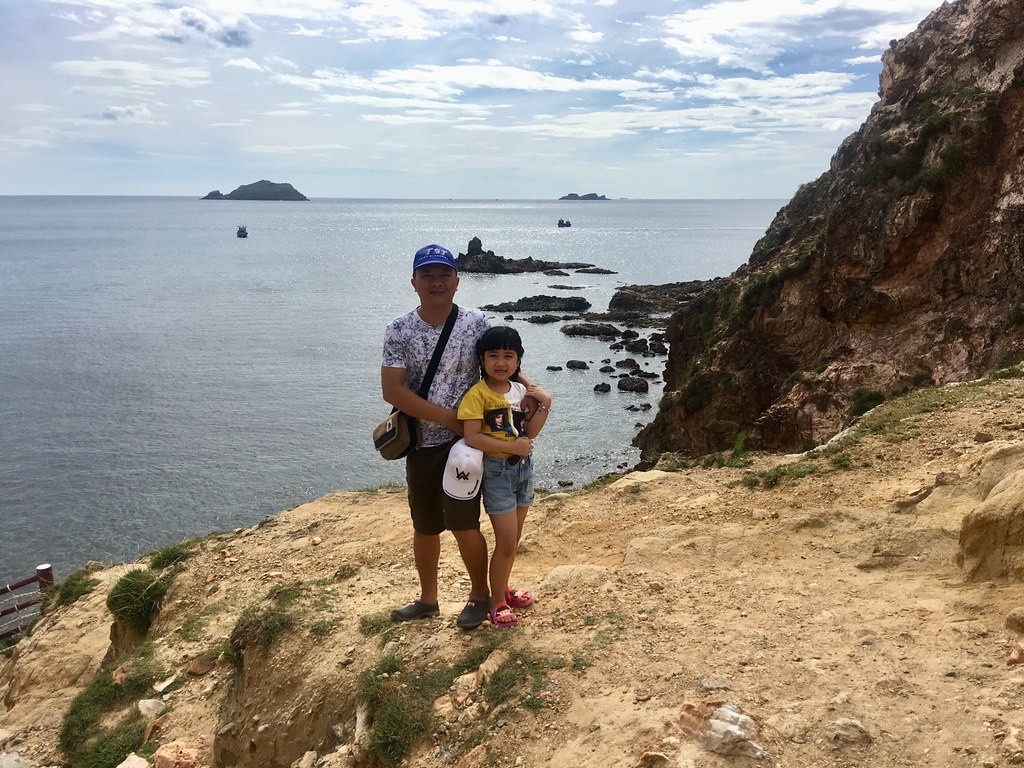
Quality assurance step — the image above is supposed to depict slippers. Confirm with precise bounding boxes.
[505,588,533,607]
[489,602,517,626]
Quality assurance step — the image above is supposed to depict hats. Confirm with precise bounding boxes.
[442,437,484,500]
[412,244,457,278]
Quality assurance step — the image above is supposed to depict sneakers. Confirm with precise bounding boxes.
[456,591,491,627]
[391,601,440,621]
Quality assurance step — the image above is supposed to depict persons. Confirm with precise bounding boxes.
[457,325,552,625]
[380,244,539,629]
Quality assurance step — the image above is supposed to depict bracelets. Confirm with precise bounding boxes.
[538,403,551,412]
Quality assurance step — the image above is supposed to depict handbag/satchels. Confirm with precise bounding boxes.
[373,409,422,460]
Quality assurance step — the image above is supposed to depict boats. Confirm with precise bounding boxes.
[565,221,571,227]
[557,219,566,227]
[237,224,248,238]
